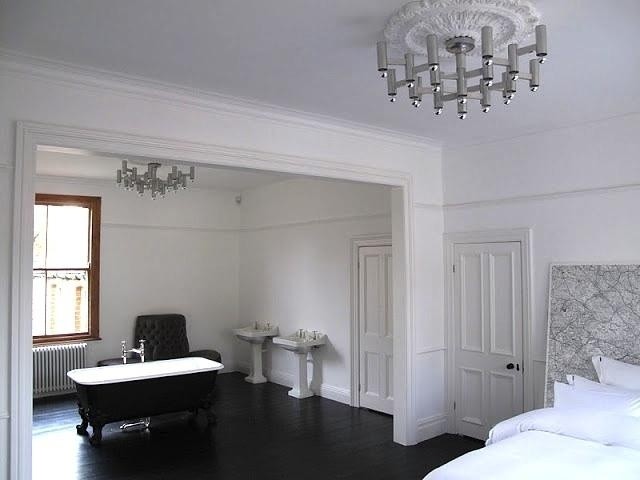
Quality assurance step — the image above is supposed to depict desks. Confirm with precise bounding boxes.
[233,327,326,401]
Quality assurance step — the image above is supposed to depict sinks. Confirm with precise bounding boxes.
[272,331,327,353]
[232,326,278,344]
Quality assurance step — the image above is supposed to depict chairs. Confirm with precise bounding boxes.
[136,312,222,398]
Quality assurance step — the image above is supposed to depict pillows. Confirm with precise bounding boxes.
[551,353,640,421]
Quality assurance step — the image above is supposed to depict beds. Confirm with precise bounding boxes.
[428,261,640,479]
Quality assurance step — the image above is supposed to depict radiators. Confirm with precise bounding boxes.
[31,342,88,399]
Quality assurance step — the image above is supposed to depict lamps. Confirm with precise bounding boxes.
[377,0,550,124]
[115,160,195,202]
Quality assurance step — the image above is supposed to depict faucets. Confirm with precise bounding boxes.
[263,323,270,333]
[121,340,128,364]
[139,340,146,361]
[309,330,317,340]
